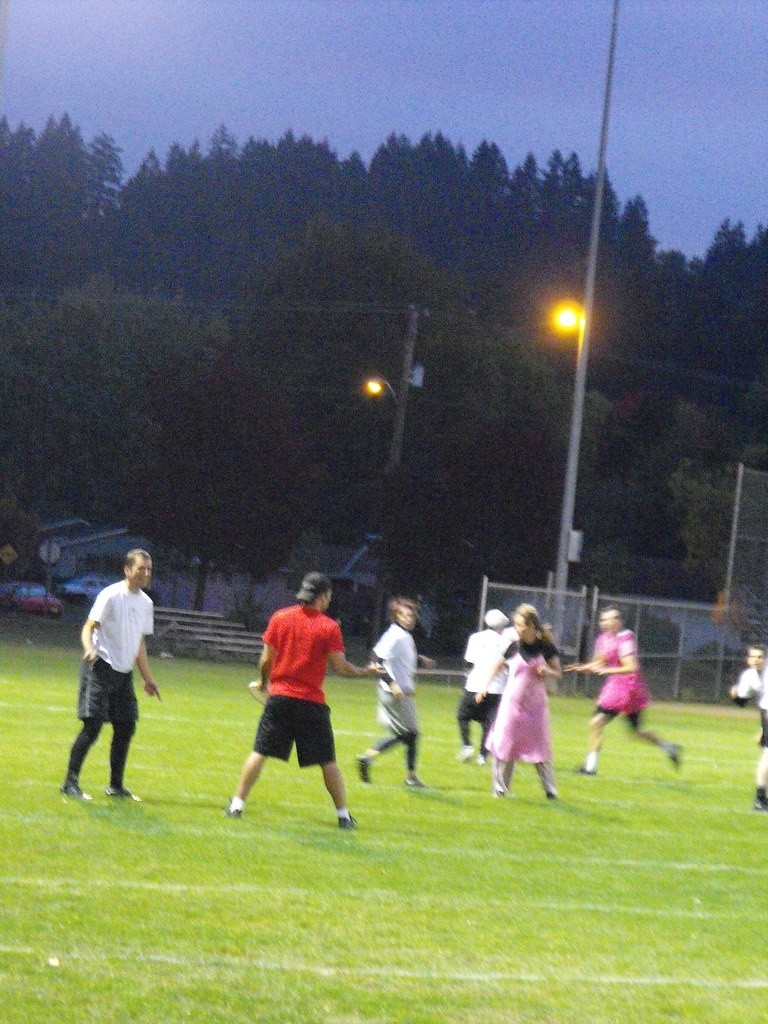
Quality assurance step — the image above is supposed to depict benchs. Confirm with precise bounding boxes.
[152,606,265,664]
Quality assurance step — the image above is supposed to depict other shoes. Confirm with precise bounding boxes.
[668,744,680,770]
[570,767,596,776]
[458,745,476,763]
[224,807,242,819]
[339,811,358,830]
[754,787,768,812]
[477,754,487,765]
[405,776,425,788]
[60,784,93,801]
[106,786,143,802]
[356,754,371,783]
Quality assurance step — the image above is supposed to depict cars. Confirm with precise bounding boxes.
[0,580,65,615]
[54,575,108,601]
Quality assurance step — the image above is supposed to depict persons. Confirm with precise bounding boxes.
[61,548,162,802]
[564,605,683,777]
[458,608,519,766]
[357,596,438,788]
[225,572,387,829]
[730,644,768,812]
[476,604,561,802]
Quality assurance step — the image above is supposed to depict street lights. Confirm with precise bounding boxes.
[553,305,590,650]
[366,377,408,663]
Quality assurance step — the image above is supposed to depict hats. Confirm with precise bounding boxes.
[485,609,510,628]
[295,571,332,602]
